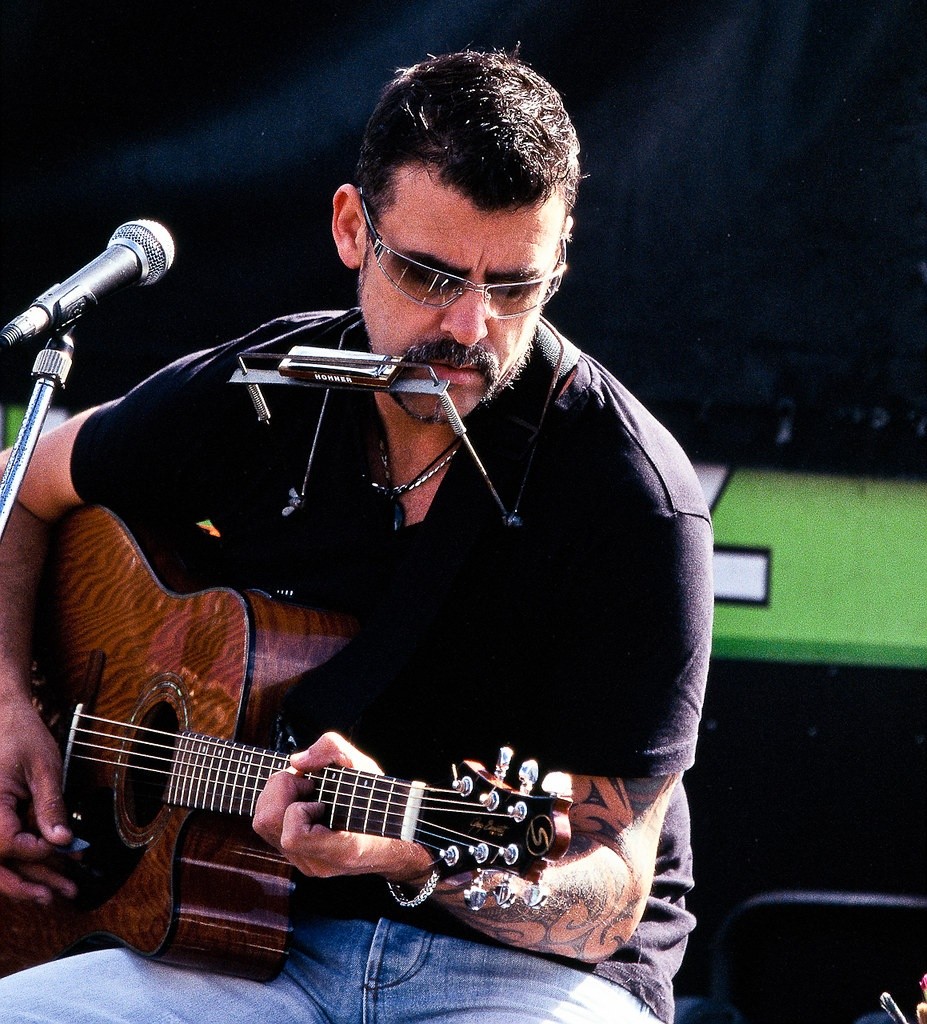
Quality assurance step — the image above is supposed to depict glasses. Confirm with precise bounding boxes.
[356,186,570,320]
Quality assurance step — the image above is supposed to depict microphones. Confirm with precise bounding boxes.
[0,220,174,350]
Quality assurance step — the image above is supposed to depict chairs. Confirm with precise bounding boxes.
[713,888,926,1024]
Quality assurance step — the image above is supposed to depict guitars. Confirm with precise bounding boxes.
[18,507,572,983]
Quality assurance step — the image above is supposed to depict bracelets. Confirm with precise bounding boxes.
[385,871,441,908]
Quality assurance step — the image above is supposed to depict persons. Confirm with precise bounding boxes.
[0,51,712,1022]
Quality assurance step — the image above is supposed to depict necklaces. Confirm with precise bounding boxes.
[371,447,458,497]
[392,435,461,496]
